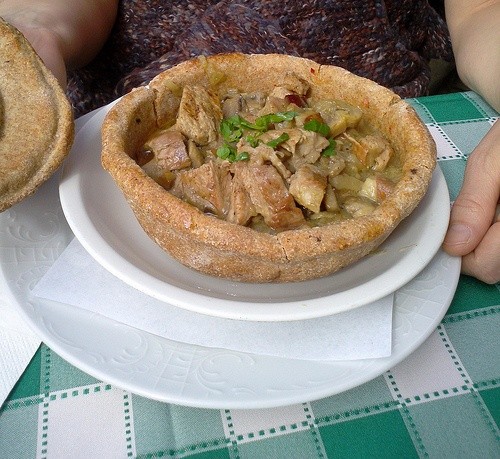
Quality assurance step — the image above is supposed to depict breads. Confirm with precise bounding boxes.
[99,51,439,282]
[0,15,73,215]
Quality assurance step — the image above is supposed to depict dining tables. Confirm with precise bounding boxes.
[0,93,500,459]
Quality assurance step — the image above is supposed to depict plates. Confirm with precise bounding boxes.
[59,86,451,320]
[0,105,462,409]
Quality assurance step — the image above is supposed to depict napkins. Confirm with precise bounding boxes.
[30,235,394,361]
[0,272,43,412]
